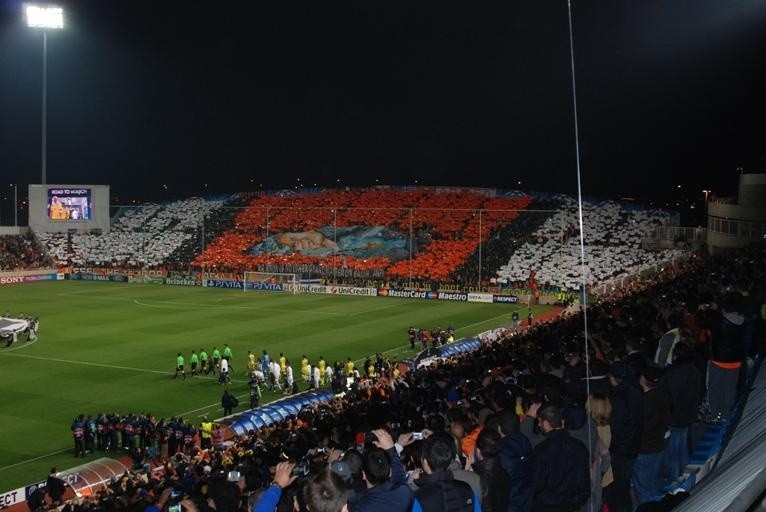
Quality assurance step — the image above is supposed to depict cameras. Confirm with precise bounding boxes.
[228,470,240,481]
[365,433,377,444]
[171,490,184,498]
[470,396,477,400]
[293,465,306,475]
[166,504,185,512]
[412,432,423,441]
[456,400,462,406]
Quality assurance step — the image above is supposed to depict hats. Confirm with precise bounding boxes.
[611,361,629,379]
[332,461,351,480]
[640,359,661,383]
[566,405,586,429]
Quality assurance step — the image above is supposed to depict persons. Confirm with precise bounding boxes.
[30,411,404,512]
[2,311,41,348]
[405,243,765,511]
[174,342,404,411]
[0,186,673,287]
